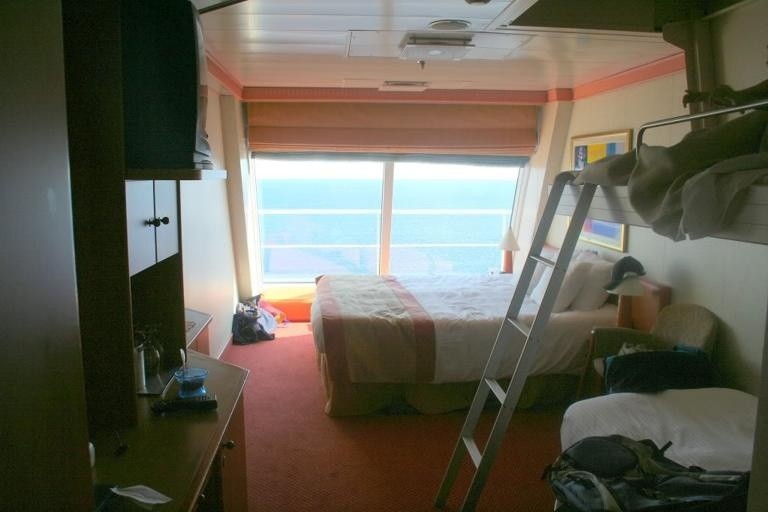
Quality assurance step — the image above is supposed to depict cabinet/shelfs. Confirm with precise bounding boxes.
[124,180,180,276]
[218,396,249,511]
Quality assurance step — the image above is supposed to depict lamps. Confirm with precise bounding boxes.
[399,33,476,64]
[498,230,520,270]
[610,278,643,326]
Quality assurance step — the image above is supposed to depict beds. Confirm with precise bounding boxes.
[312,272,620,418]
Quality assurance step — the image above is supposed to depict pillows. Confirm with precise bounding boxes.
[573,252,613,315]
[532,257,589,315]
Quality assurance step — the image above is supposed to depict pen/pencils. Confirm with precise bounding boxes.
[96,485,119,512]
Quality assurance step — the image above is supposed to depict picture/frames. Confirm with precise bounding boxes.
[566,128,632,254]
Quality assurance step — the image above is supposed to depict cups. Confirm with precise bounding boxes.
[174,368,208,398]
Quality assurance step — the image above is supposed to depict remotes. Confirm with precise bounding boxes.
[150,393,218,414]
